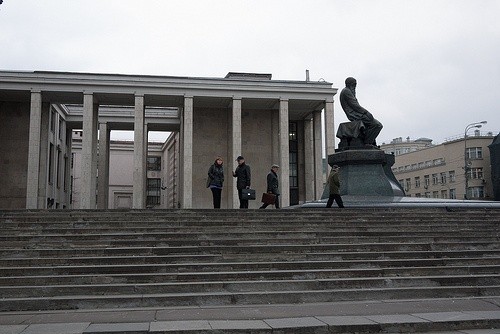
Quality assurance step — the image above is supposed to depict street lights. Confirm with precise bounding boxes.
[465,120,487,199]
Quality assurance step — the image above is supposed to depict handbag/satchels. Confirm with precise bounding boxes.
[206,176,211,188]
[242,186,256,200]
[261,192,276,205]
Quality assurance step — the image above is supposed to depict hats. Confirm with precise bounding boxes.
[333,165,340,169]
[272,164,279,168]
[235,156,244,161]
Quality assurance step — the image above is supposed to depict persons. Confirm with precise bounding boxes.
[258,163,281,209]
[339,77,383,150]
[326,165,345,208]
[208,156,225,208]
[232,155,252,208]
